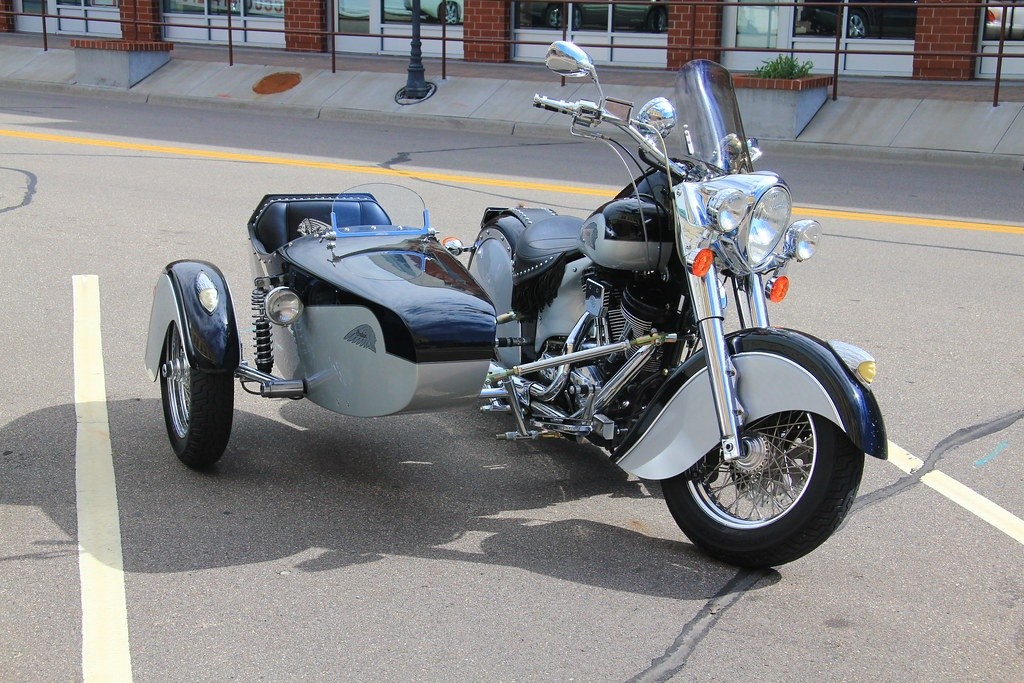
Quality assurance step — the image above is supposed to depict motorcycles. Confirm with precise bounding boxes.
[142,40,890,568]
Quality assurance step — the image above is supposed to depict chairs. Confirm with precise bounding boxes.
[246,192,392,281]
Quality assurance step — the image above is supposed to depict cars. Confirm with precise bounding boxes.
[986,7,1024,38]
[522,0,667,32]
[808,0,885,39]
[405,0,463,25]
